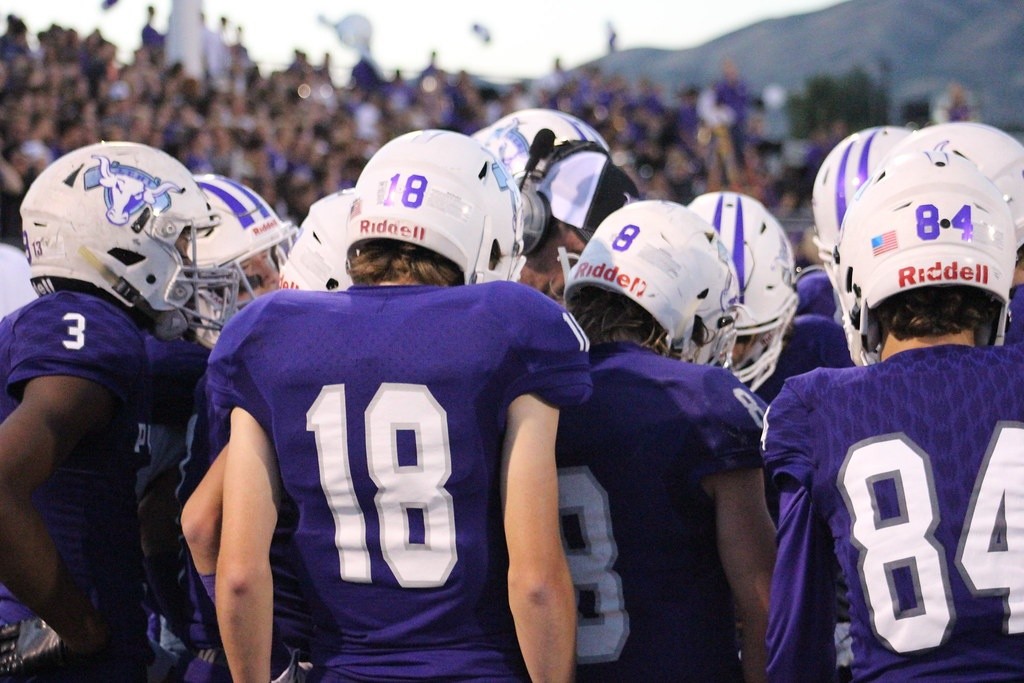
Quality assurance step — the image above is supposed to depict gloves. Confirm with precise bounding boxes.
[0,619,88,679]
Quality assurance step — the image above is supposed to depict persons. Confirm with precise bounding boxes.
[0,5,1024,683]
[205,131,591,683]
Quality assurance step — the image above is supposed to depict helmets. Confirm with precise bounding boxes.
[813,126,913,290]
[562,199,732,364]
[345,129,528,286]
[833,147,1018,334]
[685,191,799,329]
[875,121,1024,252]
[20,141,212,308]
[281,187,353,293]
[533,151,640,230]
[185,172,287,276]
[469,109,609,174]
